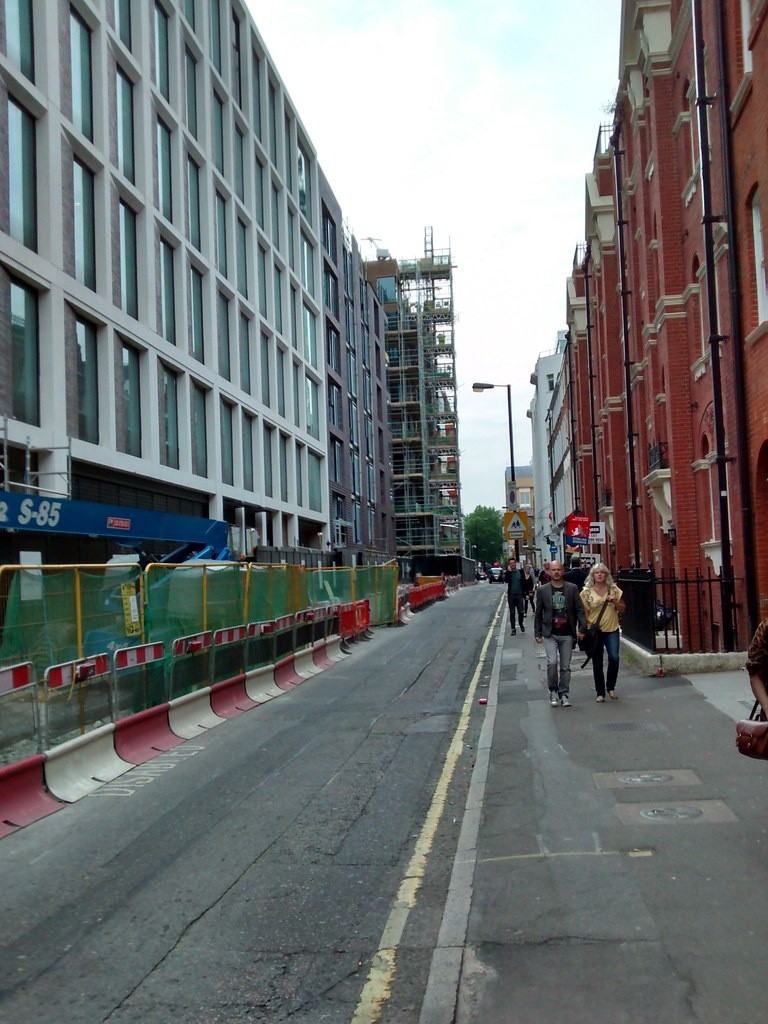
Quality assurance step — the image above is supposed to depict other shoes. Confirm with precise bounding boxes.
[520,624,525,632]
[549,690,558,707]
[510,629,516,635]
[524,614,527,617]
[561,696,571,707]
[532,612,535,616]
[597,697,607,702]
[610,691,618,699]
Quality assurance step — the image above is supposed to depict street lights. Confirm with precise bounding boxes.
[472,381,520,563]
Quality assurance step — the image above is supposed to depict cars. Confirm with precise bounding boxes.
[477,568,506,584]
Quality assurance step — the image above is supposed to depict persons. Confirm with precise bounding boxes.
[534,560,588,707]
[580,562,625,702]
[745,619,768,719]
[502,552,588,636]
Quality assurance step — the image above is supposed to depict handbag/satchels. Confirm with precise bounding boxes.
[735,719,768,760]
[579,626,603,656]
